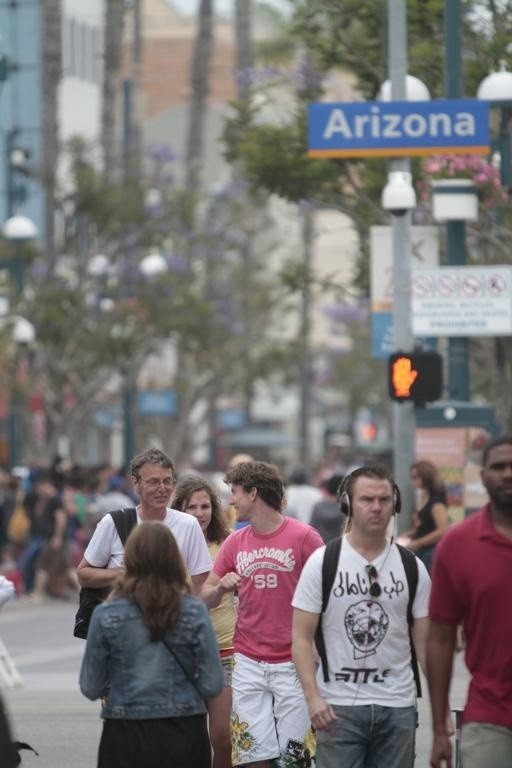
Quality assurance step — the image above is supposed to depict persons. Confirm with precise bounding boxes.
[2,437,511,768]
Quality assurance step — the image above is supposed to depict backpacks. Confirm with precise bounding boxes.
[73,505,142,638]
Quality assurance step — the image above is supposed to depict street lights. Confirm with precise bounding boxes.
[1,120,41,472]
[85,246,167,472]
[373,70,512,405]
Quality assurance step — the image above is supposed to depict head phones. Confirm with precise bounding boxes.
[337,466,401,516]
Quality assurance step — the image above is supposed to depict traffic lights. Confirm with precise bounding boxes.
[388,352,443,402]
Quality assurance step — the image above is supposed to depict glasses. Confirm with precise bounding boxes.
[138,476,178,487]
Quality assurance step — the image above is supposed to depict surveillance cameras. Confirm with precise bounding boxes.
[380,172,416,216]
